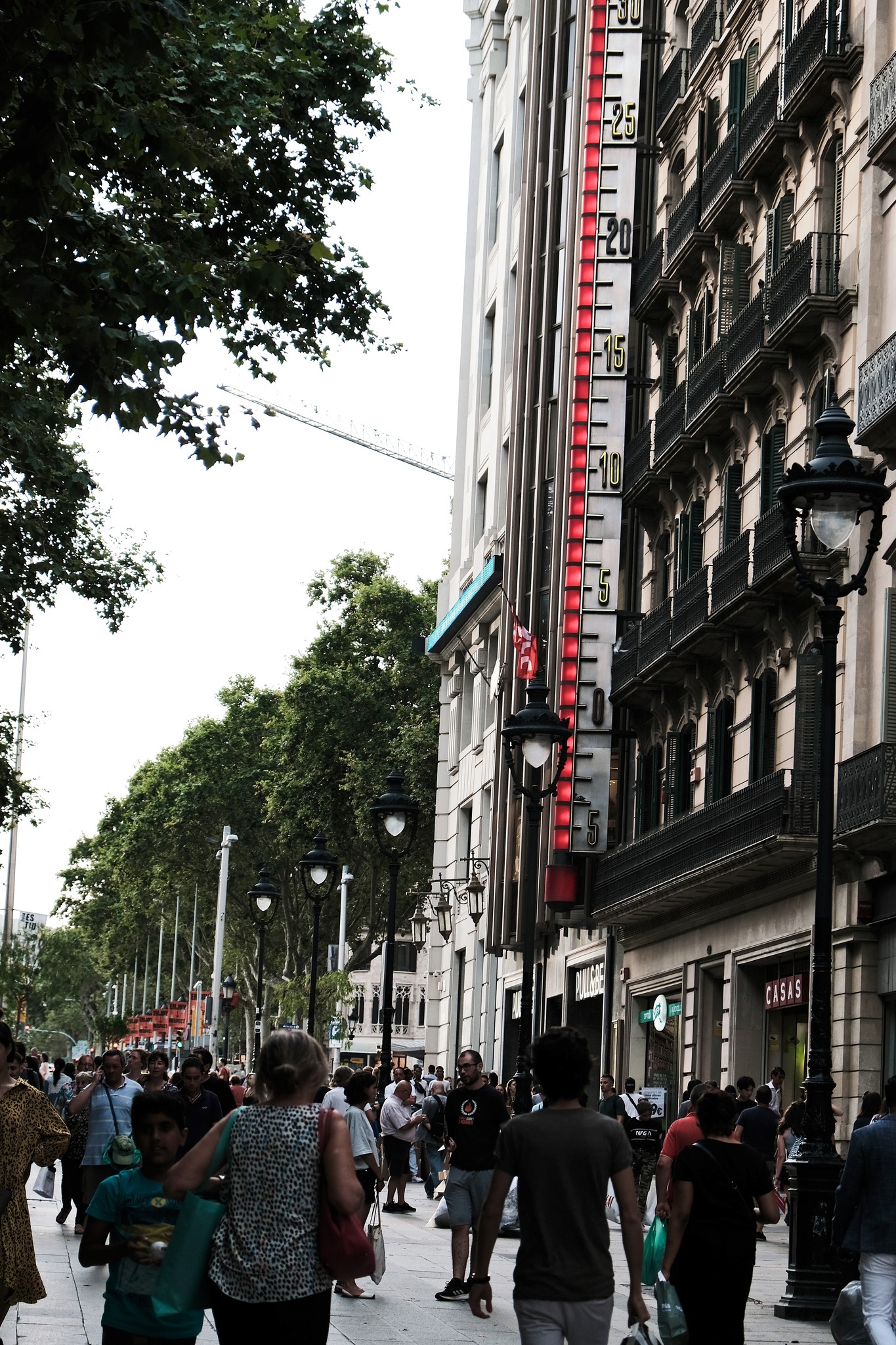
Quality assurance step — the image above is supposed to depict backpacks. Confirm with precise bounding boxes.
[223,1068,230,1083]
[431,1094,445,1137]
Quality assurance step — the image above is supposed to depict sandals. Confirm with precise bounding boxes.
[334,1285,344,1293]
[341,1289,375,1299]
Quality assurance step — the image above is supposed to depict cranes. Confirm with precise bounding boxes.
[220,374,457,484]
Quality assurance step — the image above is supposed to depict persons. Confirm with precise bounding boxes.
[380,1025,896,1345]
[0,1016,386,1345]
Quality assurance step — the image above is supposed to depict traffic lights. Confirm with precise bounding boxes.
[176,1029,184,1048]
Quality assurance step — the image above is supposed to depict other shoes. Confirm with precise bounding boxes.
[74,1224,84,1234]
[434,1194,443,1200]
[756,1231,767,1241]
[56,1206,72,1224]
[427,1195,434,1200]
[641,1223,646,1232]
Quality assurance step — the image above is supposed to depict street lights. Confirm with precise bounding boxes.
[499,681,576,1244]
[365,764,425,1145]
[220,973,238,1067]
[296,826,341,1042]
[785,362,892,1329]
[247,868,282,1081]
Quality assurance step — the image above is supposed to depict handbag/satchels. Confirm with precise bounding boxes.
[413,1079,425,1094]
[638,1174,659,1227]
[368,1192,386,1285]
[654,1270,688,1345]
[829,1280,872,1345]
[316,1107,376,1279]
[774,1190,786,1215]
[641,1213,668,1287]
[604,1178,622,1225]
[32,1166,55,1199]
[425,1198,451,1228]
[146,1106,249,1323]
[631,1158,641,1177]
[102,1145,141,1172]
[621,1322,662,1345]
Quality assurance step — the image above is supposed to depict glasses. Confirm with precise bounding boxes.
[456,1063,479,1072]
[776,1076,785,1080]
[403,1089,411,1096]
[247,1073,256,1080]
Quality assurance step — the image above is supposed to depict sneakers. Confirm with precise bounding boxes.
[394,1202,416,1213]
[382,1199,405,1213]
[435,1277,470,1300]
[411,1175,423,1183]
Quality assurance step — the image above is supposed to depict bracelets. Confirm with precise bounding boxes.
[378,1177,384,1182]
[774,1176,780,1180]
[471,1273,491,1285]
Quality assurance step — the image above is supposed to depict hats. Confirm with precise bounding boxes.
[625,1077,635,1092]
[111,1134,136,1165]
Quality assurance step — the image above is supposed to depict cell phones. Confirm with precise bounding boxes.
[99,1066,105,1083]
